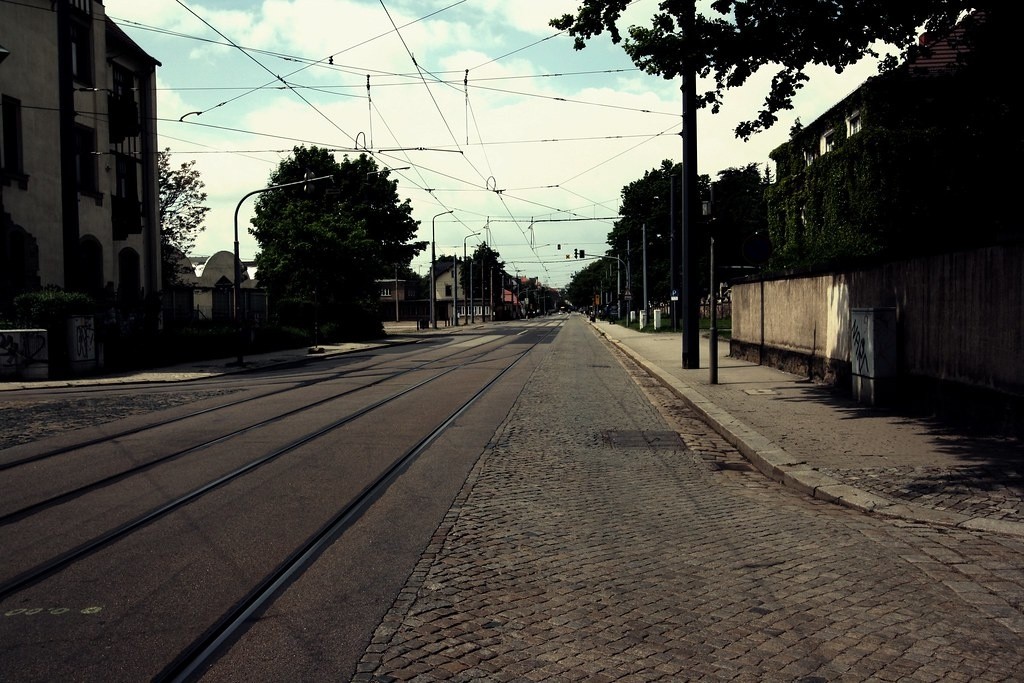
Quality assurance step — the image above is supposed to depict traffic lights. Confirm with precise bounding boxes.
[574,248,577,259]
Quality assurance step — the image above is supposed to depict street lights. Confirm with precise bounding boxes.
[233,172,333,366]
[431,210,453,329]
[464,232,481,324]
